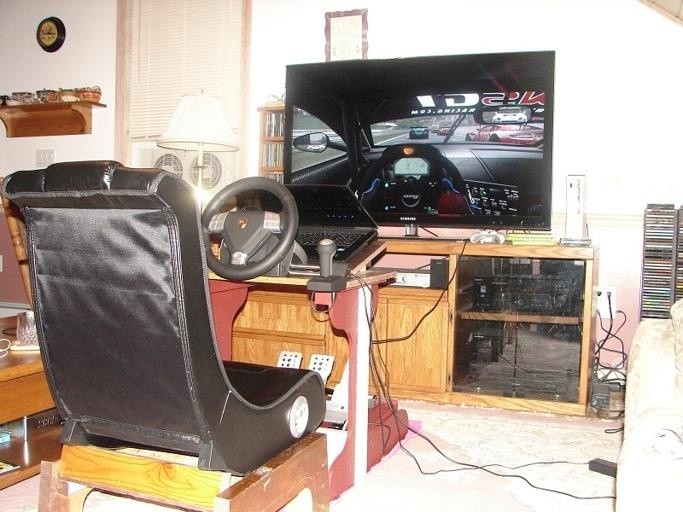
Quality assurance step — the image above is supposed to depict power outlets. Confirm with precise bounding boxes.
[596,288,616,319]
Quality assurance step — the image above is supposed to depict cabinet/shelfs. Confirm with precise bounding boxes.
[230,236,599,417]
[258,104,285,187]
[637,204,683,320]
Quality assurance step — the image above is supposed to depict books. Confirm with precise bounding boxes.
[505,232,591,248]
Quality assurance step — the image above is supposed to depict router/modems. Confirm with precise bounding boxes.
[564,175,589,244]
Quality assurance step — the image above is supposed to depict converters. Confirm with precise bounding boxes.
[592,383,611,407]
[589,456,617,479]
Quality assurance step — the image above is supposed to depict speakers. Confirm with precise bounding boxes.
[429,259,446,289]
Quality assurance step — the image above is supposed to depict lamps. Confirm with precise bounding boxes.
[155,96,241,209]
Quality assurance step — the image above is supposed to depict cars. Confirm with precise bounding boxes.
[306,145,324,152]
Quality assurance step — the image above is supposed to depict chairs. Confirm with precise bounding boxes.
[0,160,331,512]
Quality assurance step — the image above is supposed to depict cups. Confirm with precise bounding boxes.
[10,88,101,104]
[16,311,39,345]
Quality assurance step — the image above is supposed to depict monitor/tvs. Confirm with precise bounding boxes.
[279,49,555,241]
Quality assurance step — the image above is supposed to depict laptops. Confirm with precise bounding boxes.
[276,184,378,266]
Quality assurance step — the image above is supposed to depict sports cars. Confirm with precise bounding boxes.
[407,106,544,148]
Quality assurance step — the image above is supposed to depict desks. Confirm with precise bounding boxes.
[207,268,406,502]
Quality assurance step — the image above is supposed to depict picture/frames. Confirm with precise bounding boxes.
[324,7,368,61]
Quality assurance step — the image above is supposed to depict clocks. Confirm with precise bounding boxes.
[37,17,66,52]
